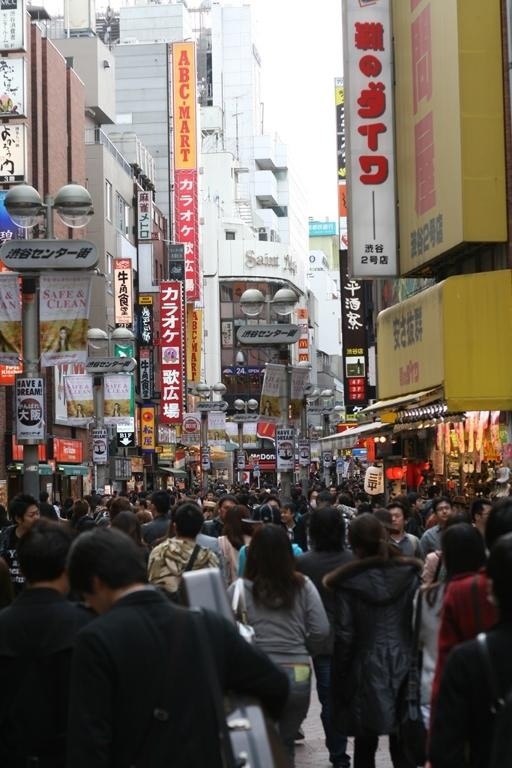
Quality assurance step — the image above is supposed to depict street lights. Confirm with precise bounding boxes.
[0,184,99,502]
[85,323,135,491]
[232,288,334,502]
[193,382,229,493]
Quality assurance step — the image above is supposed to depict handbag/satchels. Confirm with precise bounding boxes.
[390,663,424,767]
[219,704,294,768]
[161,584,188,605]
[234,620,257,647]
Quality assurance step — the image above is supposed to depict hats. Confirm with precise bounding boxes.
[203,500,216,507]
[453,496,465,503]
[241,504,281,529]
[375,508,401,534]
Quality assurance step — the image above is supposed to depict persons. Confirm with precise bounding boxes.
[109,403,124,416]
[0,467,512,768]
[73,404,85,418]
[54,326,75,352]
[262,401,274,416]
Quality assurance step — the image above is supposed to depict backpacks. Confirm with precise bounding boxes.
[471,689,512,767]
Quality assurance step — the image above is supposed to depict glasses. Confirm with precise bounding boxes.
[203,507,214,513]
[76,600,95,611]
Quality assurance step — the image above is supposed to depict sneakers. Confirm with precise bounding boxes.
[294,727,305,745]
